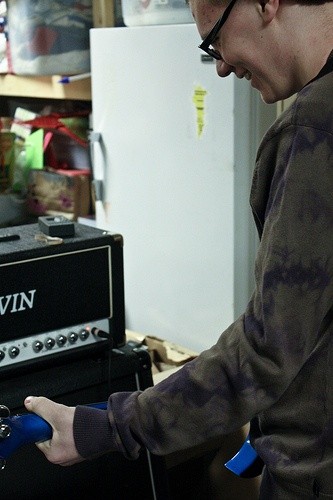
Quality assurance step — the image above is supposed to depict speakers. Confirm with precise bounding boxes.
[0,341,173,500]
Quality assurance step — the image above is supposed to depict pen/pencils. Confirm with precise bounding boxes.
[57,71,91,84]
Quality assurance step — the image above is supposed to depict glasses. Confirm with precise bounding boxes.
[198,0,237,60]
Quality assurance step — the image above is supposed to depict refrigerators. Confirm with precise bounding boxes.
[87,23,277,356]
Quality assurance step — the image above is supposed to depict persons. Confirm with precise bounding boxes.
[24,0,333,500]
